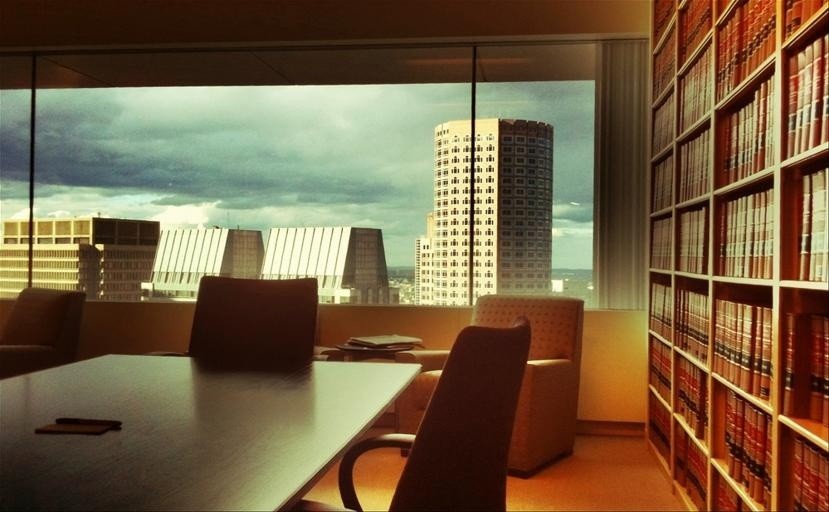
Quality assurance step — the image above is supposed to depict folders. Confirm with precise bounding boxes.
[346,334,423,347]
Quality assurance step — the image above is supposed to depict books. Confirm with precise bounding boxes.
[650,0,829,512]
[336,332,426,351]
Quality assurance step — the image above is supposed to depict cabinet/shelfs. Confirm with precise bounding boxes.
[643,0,829,512]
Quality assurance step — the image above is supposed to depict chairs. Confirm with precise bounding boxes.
[188,275,319,361]
[1,286,90,378]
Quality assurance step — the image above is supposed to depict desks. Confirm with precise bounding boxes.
[1,354,423,512]
